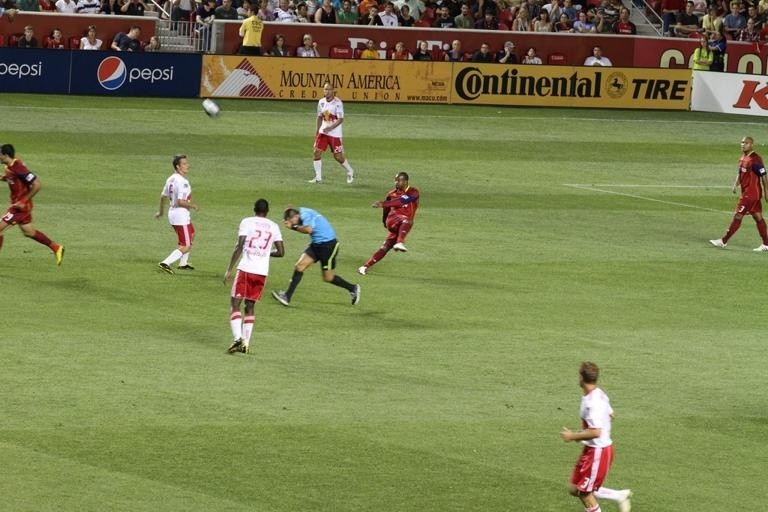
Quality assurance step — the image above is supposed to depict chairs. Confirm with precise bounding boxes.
[0,0,767,71]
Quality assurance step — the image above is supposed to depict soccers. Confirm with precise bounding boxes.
[201,98,220,119]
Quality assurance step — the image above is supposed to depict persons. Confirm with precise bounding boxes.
[660,0,768,72]
[584,46,612,66]
[239,5,264,56]
[0,143,66,266]
[308,83,356,184]
[237,0,638,35]
[560,361,635,512]
[272,206,363,306]
[154,154,199,275]
[708,137,768,252]
[152,1,238,52]
[1,1,159,52]
[358,172,420,276]
[222,198,285,354]
[268,33,544,65]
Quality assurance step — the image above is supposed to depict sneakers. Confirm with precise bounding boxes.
[156,262,176,276]
[306,176,322,185]
[753,244,768,252]
[708,238,727,249]
[228,337,250,354]
[393,242,407,252]
[357,265,368,276]
[270,290,290,306]
[349,283,361,306]
[56,244,65,266]
[176,264,195,270]
[346,169,354,184]
[617,488,633,512]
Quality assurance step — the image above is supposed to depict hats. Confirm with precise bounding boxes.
[504,41,516,49]
